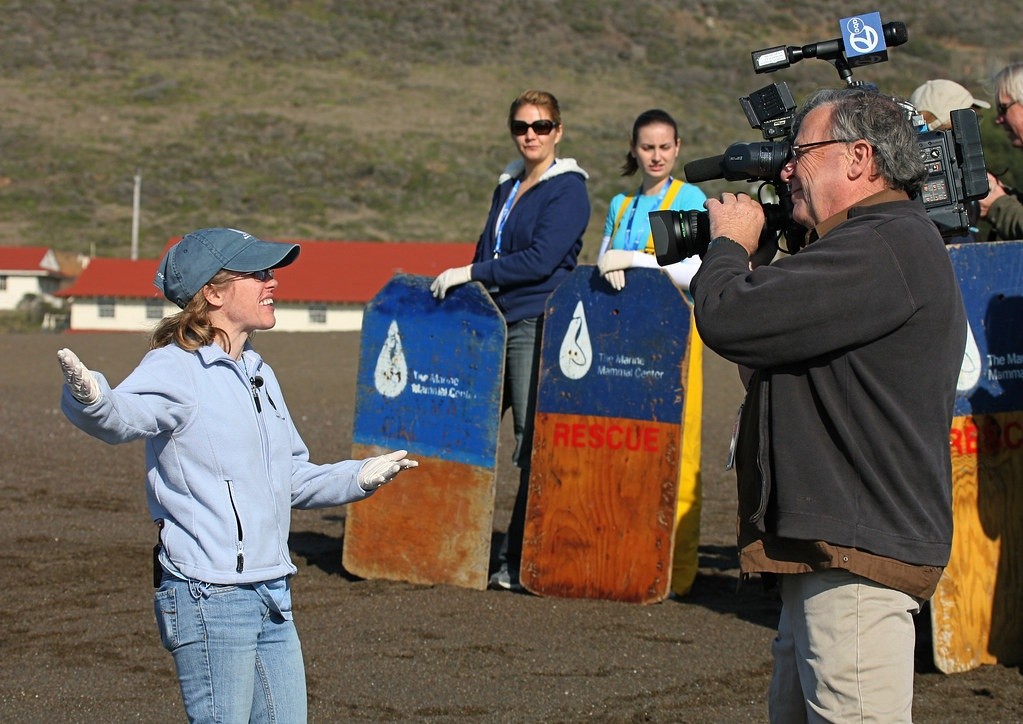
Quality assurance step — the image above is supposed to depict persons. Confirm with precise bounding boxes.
[596,108,710,291]
[908,63,1023,241]
[694,89,967,723]
[430,89,591,591]
[56,228,418,724]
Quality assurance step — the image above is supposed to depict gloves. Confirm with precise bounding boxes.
[430,263,472,298]
[56,347,102,406]
[357,449,418,491]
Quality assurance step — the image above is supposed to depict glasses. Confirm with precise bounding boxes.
[997,99,1020,115]
[218,268,273,283]
[511,119,557,135]
[790,139,877,158]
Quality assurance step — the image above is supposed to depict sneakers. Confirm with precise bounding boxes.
[491,568,523,590]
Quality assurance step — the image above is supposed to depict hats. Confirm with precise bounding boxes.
[153,226,300,310]
[910,79,991,131]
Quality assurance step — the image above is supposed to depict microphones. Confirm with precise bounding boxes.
[684,154,726,183]
[254,376,263,388]
[751,12,908,74]
